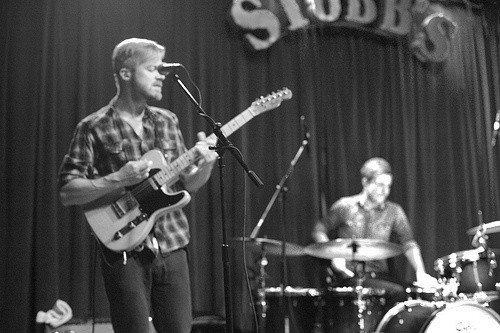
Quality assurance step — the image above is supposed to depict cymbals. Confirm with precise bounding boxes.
[301,236,413,262]
[219,237,308,257]
[465,221,500,235]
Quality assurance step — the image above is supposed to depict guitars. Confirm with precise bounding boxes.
[81,86,293,254]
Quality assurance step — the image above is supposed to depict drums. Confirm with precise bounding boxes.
[374,299,500,333]
[251,285,326,333]
[405,288,437,303]
[433,246,500,305]
[322,286,392,333]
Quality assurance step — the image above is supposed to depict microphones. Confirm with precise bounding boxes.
[157,62,182,74]
[301,117,312,157]
[491,110,500,146]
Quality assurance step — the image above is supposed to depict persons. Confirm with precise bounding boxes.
[311,157,438,312]
[56,38,219,333]
